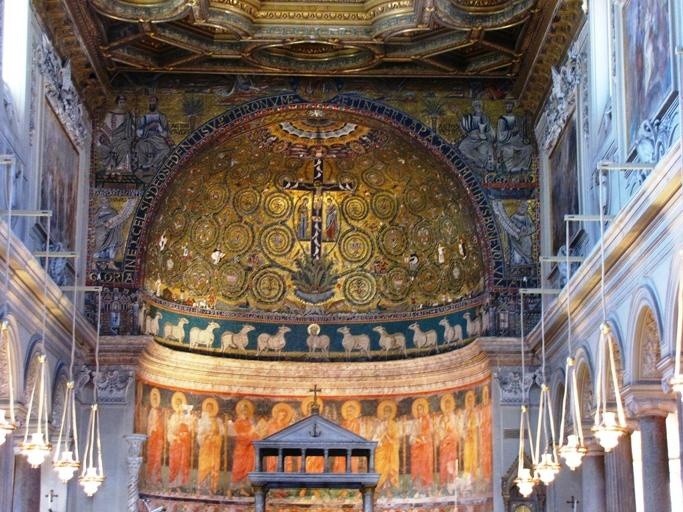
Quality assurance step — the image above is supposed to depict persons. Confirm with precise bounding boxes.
[192,396,225,500]
[298,177,338,218]
[497,99,536,174]
[88,194,127,272]
[93,93,136,178]
[329,396,364,503]
[298,393,326,499]
[370,398,403,500]
[293,196,311,241]
[262,402,297,499]
[475,384,494,482]
[447,97,495,167]
[131,95,173,174]
[407,396,434,497]
[461,390,479,480]
[324,196,339,240]
[506,200,538,267]
[143,386,165,490]
[223,399,260,499]
[436,390,459,498]
[164,390,195,497]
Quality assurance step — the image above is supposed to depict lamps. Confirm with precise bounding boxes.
[0,153,107,499]
[514,161,630,500]
